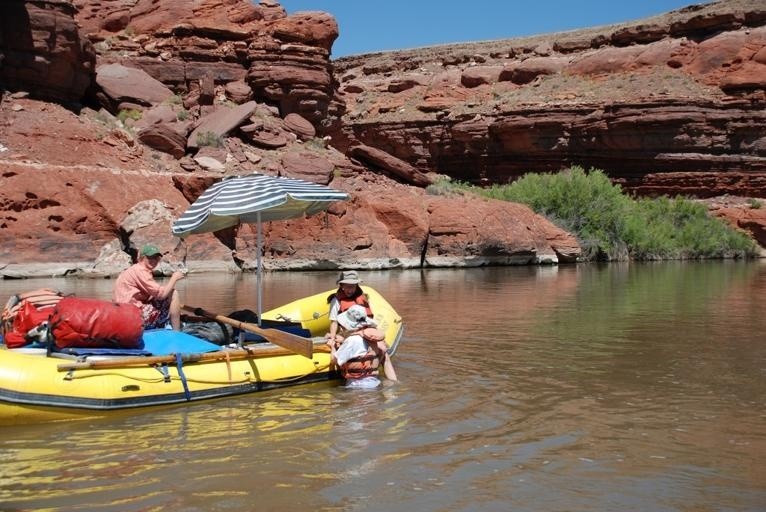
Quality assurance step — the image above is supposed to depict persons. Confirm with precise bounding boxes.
[113,244,187,334]
[326,304,383,391]
[326,269,400,384]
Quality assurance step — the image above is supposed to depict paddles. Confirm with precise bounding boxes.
[178,304,314,358]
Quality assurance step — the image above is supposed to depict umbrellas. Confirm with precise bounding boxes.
[173,170,348,329]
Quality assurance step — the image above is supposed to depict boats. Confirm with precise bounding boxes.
[0,382,411,512]
[0,287,405,412]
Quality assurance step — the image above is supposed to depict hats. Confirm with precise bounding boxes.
[337,304,377,330]
[337,271,361,285]
[140,245,163,259]
